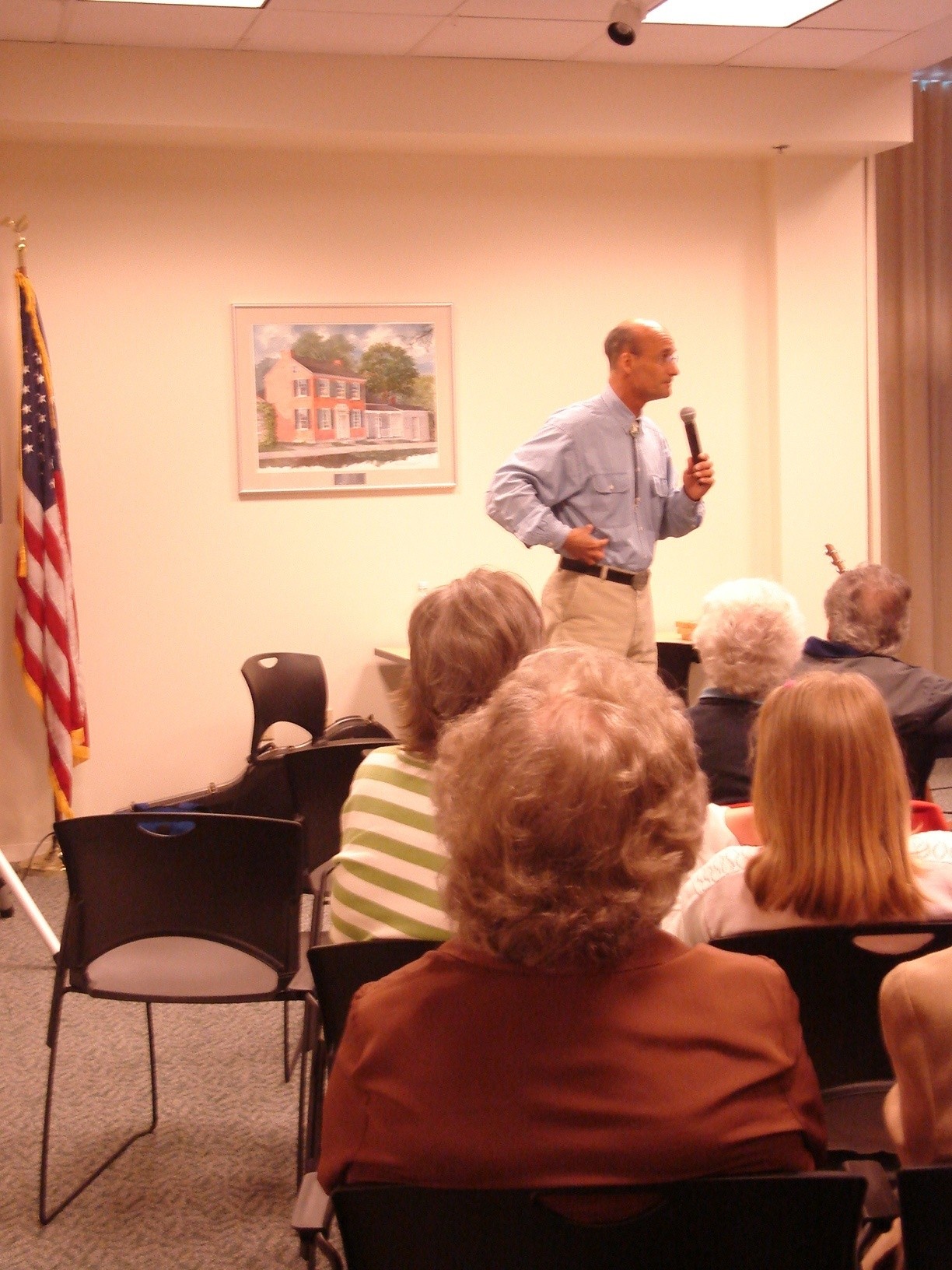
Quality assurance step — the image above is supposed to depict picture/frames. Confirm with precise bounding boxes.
[230,303,458,495]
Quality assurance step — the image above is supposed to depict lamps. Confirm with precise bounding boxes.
[607,0,646,46]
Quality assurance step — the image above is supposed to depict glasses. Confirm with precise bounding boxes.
[635,354,681,364]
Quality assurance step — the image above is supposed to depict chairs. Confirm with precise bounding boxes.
[39,652,952,1270]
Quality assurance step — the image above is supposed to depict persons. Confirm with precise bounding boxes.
[314,643,829,1185]
[787,564,951,800]
[681,579,802,806]
[664,666,952,961]
[330,567,542,942]
[484,320,715,683]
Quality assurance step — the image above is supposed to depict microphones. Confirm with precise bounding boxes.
[681,406,701,463]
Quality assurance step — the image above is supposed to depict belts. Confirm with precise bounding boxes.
[559,558,649,592]
